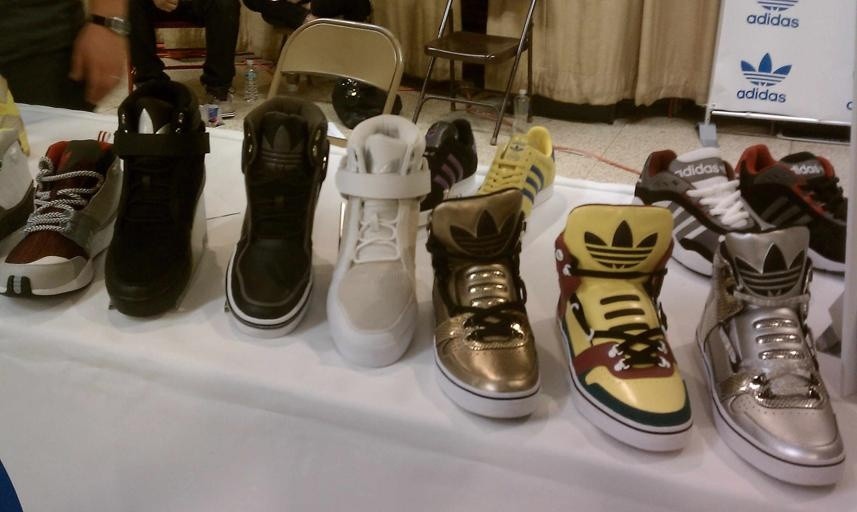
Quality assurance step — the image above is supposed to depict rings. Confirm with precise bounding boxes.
[112,74,121,80]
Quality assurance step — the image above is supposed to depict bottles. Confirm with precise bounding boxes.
[243,58,261,103]
[511,87,532,137]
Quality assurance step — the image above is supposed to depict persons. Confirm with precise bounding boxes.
[242,1,372,30]
[128,0,241,119]
[0,0,127,112]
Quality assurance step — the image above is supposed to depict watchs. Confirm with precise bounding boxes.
[90,15,130,34]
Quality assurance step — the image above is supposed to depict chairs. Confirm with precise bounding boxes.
[412,0,538,146]
[265,18,405,116]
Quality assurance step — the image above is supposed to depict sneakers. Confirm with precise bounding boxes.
[553,205,694,453]
[1,130,123,299]
[225,95,330,339]
[0,76,37,238]
[476,125,555,218]
[416,118,479,229]
[326,113,433,367]
[696,224,845,487]
[631,146,761,277]
[105,77,210,318]
[424,185,541,419]
[209,89,236,118]
[733,142,846,274]
